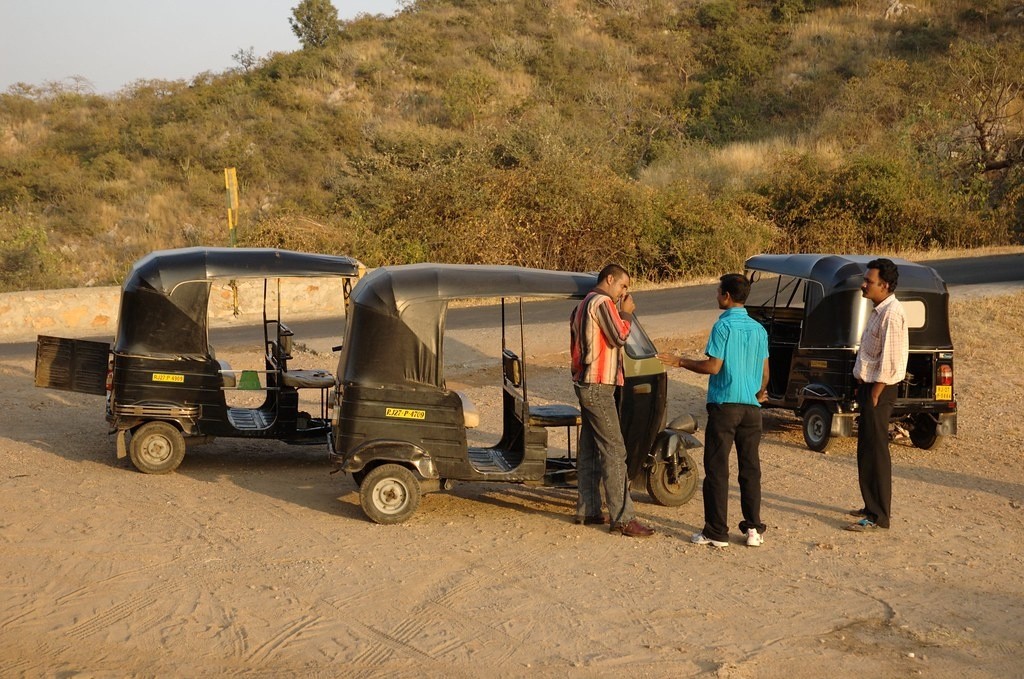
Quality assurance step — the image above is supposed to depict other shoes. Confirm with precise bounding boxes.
[846,518,891,531]
[850,509,867,517]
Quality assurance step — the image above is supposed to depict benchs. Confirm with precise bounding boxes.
[502,348,582,427]
[451,390,479,428]
[270,322,335,389]
[210,344,236,387]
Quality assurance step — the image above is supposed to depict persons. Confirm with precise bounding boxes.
[655,273,770,547]
[569,263,655,537]
[847,258,909,531]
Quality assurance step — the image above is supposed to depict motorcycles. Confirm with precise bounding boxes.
[740,254,958,453]
[326,263,707,525]
[105,246,370,475]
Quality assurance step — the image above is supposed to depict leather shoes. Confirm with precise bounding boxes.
[609,518,655,537]
[576,512,611,525]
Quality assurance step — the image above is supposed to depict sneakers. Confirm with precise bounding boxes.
[747,528,764,546]
[690,532,729,547]
